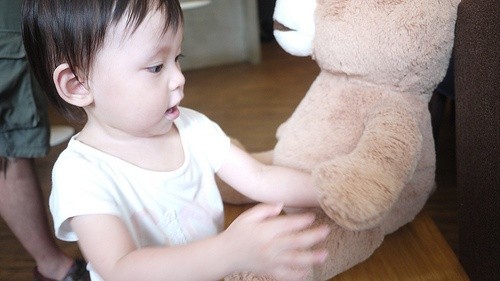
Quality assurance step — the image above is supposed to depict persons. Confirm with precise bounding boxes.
[24,1,331,281]
[0,0,84,281]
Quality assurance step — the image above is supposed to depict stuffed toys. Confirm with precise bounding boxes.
[214,0,457,281]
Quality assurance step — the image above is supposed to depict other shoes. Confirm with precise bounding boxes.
[34,257,90,281]
